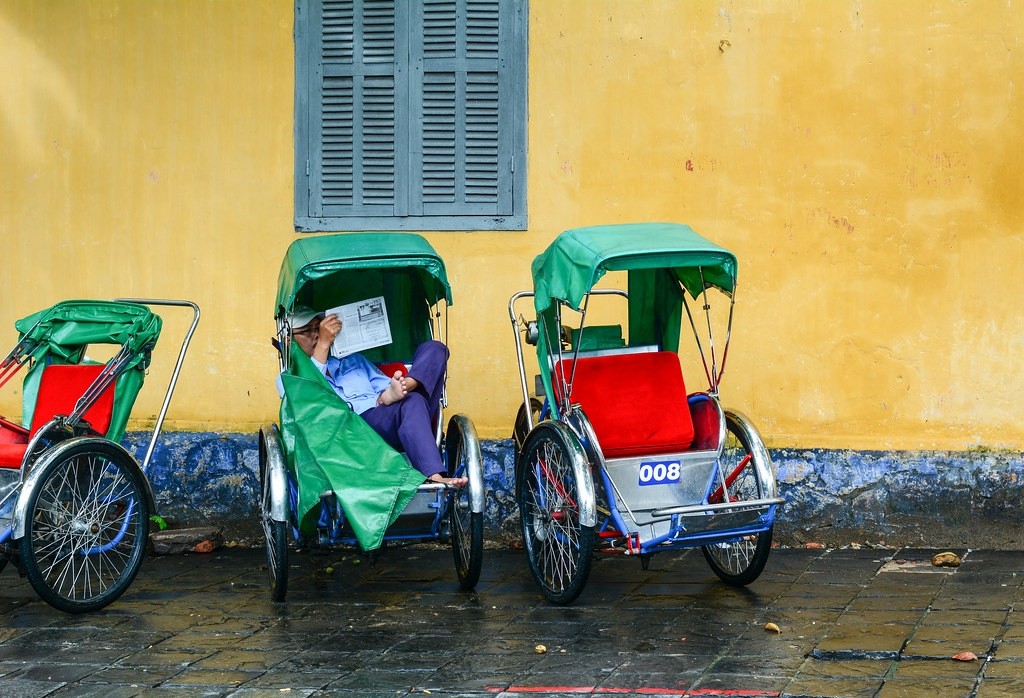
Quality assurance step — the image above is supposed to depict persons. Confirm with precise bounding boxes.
[287,306,468,484]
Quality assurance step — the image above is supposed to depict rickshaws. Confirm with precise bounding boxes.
[0,298,202,612]
[506,221,786,604]
[253,231,490,604]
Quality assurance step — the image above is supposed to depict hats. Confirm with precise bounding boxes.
[280,304,325,337]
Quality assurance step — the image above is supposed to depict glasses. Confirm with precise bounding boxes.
[292,325,320,337]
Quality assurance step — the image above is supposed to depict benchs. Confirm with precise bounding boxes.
[368,361,412,454]
[551,351,723,462]
[0,363,119,471]
